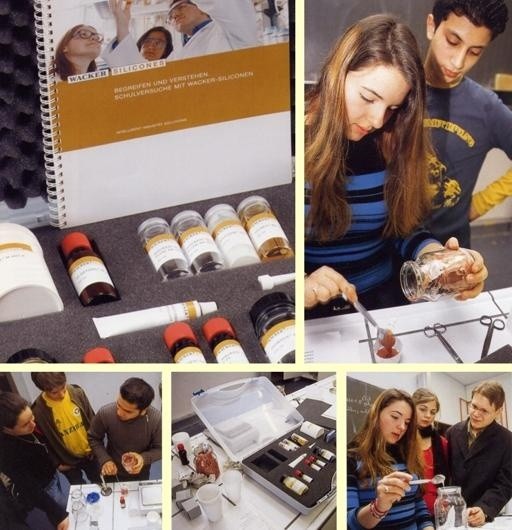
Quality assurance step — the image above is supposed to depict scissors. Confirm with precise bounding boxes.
[481,315,505,359]
[424,322,463,364]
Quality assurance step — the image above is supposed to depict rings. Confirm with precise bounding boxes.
[383,486,390,494]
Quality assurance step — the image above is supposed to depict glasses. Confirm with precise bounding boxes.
[467,402,490,418]
[141,38,166,49]
[72,29,104,43]
[165,1,196,25]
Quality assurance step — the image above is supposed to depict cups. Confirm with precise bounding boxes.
[193,444,221,479]
[196,485,225,523]
[372,337,403,363]
[223,469,242,504]
[70,489,87,508]
[71,501,89,522]
[172,431,192,465]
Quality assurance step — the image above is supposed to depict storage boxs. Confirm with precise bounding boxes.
[189,376,337,515]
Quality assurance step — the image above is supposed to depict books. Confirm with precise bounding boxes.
[30,0,294,233]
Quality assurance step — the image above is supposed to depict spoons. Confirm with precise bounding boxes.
[407,474,447,487]
[341,292,396,347]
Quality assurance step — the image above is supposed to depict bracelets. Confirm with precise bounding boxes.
[369,500,387,521]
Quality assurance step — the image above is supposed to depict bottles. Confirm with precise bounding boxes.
[313,445,336,463]
[249,291,295,364]
[308,456,327,468]
[304,459,322,472]
[279,474,309,496]
[294,469,314,484]
[434,485,467,530]
[163,322,208,365]
[59,229,120,308]
[177,443,189,466]
[203,201,261,269]
[169,208,225,273]
[1,224,64,325]
[237,194,296,262]
[137,213,190,283]
[202,316,251,363]
[399,248,478,303]
[119,495,126,510]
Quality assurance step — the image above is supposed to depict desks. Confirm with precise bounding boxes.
[171,373,337,529]
[66,479,163,530]
[305,287,512,364]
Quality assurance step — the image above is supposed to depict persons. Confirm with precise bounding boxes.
[99,0,174,76]
[303,7,490,319]
[88,376,162,485]
[444,381,511,529]
[52,22,105,82]
[347,386,436,530]
[0,395,72,510]
[409,387,450,528]
[30,372,101,485]
[1,373,21,398]
[414,1,510,250]
[0,472,70,530]
[162,0,267,66]
